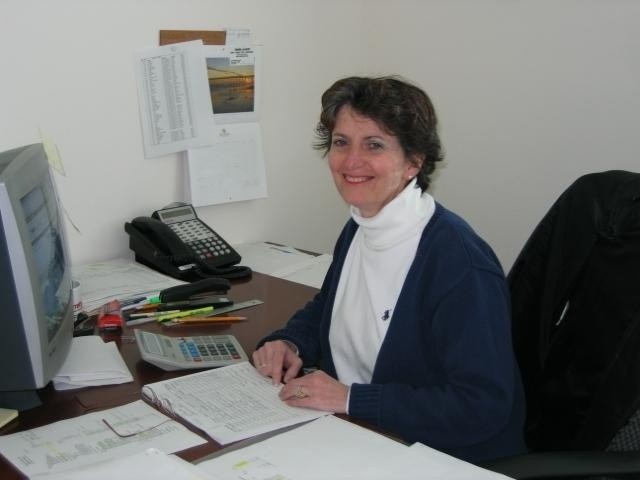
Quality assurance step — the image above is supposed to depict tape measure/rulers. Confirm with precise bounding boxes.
[161,299,264,326]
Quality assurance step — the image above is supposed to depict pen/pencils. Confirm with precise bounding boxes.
[120,296,247,326]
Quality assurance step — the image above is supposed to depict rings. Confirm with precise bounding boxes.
[259,364,265,369]
[295,384,306,398]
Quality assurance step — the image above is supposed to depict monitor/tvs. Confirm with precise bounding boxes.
[0,142,74,412]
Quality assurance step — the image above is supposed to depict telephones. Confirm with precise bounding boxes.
[125,204,241,279]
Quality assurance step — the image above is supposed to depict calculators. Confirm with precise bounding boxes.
[133,329,250,373]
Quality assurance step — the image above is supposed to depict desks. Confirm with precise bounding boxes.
[0,243,416,480]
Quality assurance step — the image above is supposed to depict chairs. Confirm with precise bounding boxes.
[481,170,640,480]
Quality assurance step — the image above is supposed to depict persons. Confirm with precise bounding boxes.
[251,76,525,465]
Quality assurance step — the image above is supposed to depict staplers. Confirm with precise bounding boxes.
[160,278,233,311]
